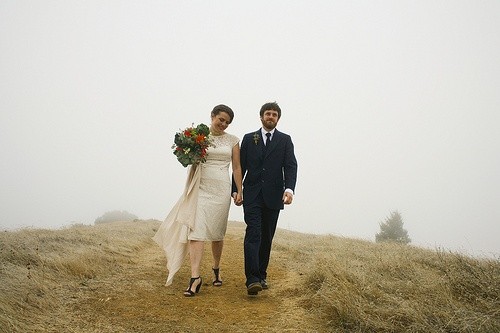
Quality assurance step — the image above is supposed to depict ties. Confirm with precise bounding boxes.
[265,133,271,146]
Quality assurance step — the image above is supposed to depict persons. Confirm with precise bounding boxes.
[231,102,297,295]
[150,103,243,297]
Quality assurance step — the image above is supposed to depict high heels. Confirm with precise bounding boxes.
[212,268,222,286]
[184,276,202,296]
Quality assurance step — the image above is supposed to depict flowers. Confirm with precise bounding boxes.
[254,131,260,144]
[171,123,215,168]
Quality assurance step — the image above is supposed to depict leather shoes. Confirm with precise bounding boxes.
[247,282,262,295]
[260,279,270,288]
